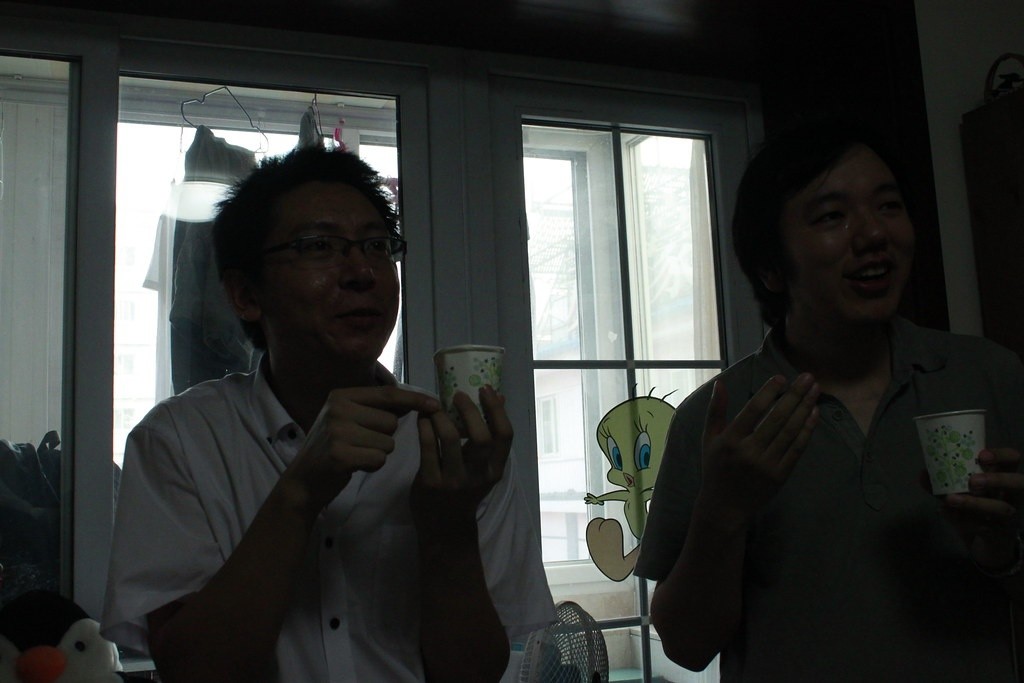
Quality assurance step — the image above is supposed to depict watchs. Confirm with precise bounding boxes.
[964,527,1024,580]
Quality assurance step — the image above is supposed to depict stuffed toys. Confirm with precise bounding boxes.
[0,591,124,683]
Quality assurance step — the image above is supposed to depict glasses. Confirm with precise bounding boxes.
[266,234,408,265]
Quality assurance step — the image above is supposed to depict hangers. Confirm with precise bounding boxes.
[181,84,269,153]
[298,92,323,138]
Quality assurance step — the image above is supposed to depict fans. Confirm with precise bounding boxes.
[519,601,609,683]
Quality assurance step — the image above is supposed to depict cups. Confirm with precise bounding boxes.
[433,343,506,437]
[912,408,992,496]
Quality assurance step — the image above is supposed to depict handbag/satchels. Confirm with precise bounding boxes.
[958,52,1024,356]
[0,432,86,564]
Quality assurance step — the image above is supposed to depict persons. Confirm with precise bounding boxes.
[631,108,1024,683]
[97,143,561,683]
